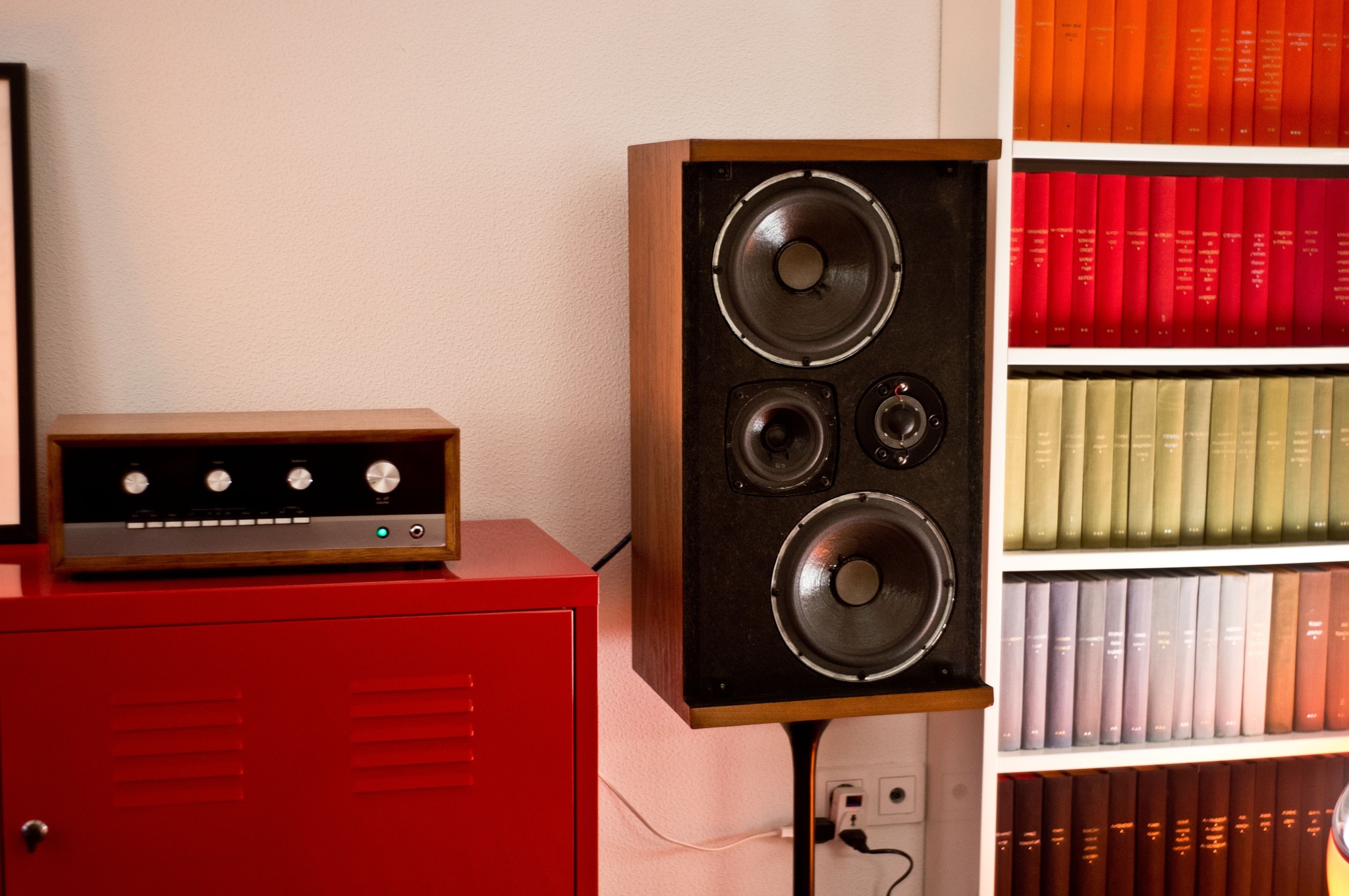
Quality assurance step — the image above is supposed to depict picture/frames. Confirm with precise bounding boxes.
[0,59,43,547]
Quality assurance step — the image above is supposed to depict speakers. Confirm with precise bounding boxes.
[623,137,1005,733]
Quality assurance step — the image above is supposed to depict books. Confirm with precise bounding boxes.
[1014,0,1349,148]
[1003,371,1348,550]
[994,752,1349,896]
[998,562,1349,750]
[1008,173,1349,347]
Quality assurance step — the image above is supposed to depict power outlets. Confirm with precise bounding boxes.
[814,765,872,842]
[865,763,927,828]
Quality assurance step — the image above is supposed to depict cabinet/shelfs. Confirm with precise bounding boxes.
[0,517,600,896]
[919,0,1349,896]
